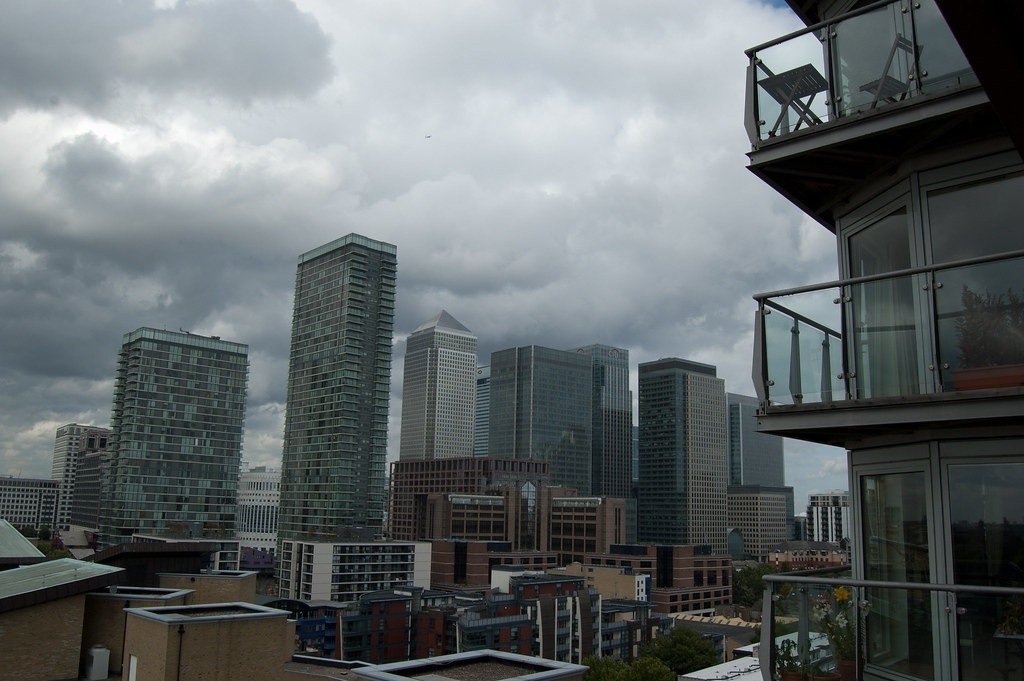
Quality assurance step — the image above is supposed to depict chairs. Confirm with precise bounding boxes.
[857,32,924,111]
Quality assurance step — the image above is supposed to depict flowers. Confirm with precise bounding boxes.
[834,587,853,602]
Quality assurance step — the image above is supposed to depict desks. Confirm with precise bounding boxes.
[756,63,833,139]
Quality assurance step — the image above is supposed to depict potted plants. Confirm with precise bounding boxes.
[774,639,807,681]
[821,615,868,681]
[804,660,842,681]
[950,283,1024,393]
